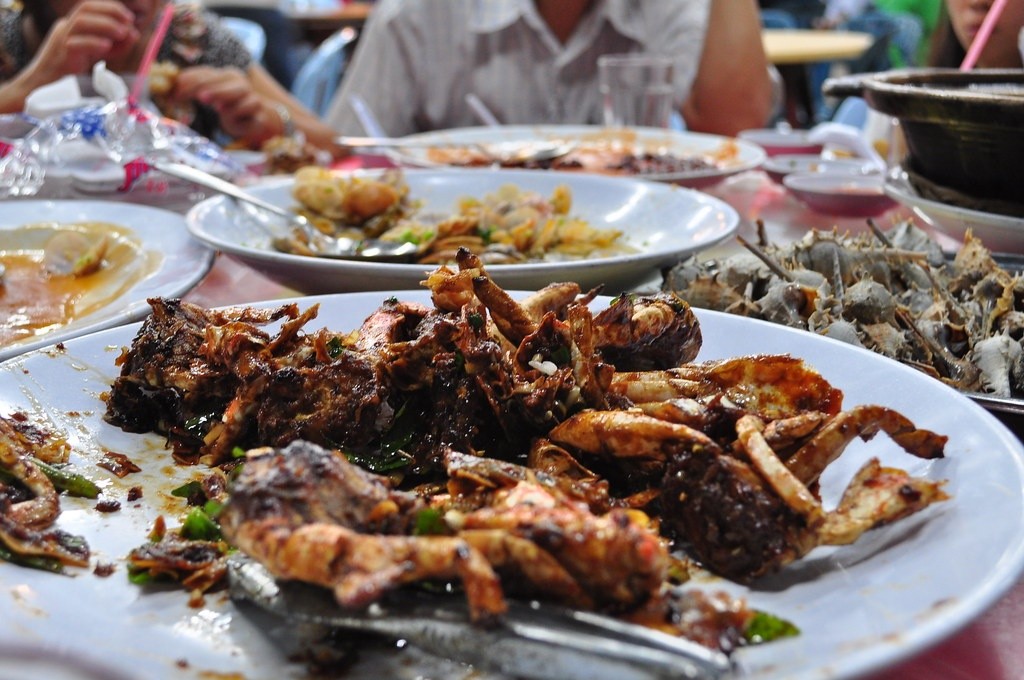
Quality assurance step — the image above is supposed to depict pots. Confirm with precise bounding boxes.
[819,66,1024,219]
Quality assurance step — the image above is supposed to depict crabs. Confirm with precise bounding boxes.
[0,248,953,628]
[273,165,623,263]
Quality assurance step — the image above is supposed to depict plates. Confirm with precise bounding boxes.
[0,291,1023,680]
[396,126,765,183]
[764,154,878,184]
[0,200,214,368]
[660,237,1023,416]
[186,170,742,294]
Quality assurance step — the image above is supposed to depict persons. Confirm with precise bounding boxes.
[925,0,1024,68]
[324,0,777,141]
[0,0,351,157]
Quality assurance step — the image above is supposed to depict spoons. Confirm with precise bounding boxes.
[331,134,577,164]
[147,155,417,256]
[230,542,702,679]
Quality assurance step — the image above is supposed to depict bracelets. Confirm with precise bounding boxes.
[268,100,293,138]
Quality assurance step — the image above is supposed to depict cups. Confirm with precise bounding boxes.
[597,54,676,127]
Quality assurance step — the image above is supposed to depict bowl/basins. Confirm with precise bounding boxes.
[885,171,1024,256]
[782,175,897,217]
